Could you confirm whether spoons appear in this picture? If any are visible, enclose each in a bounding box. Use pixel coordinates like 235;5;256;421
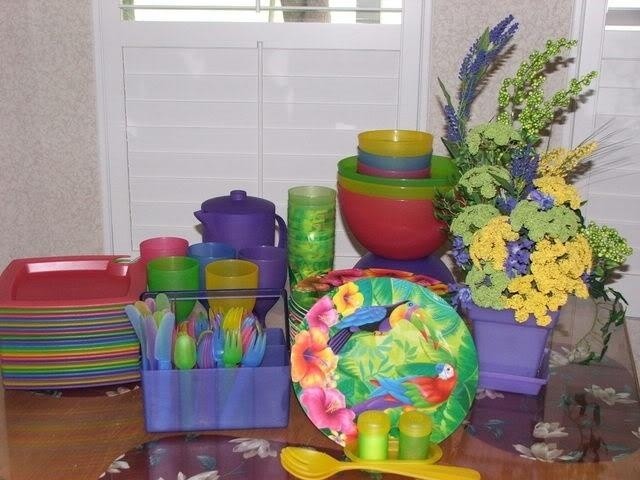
173;333;198;370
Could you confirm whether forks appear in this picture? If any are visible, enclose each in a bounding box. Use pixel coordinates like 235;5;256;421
192;307;269;369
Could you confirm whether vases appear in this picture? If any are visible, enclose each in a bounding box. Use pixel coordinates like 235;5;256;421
462;294;562;399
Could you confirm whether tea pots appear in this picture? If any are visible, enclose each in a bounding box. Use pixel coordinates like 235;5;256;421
194;189;286;247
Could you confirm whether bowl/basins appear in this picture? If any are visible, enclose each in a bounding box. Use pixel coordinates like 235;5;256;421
336;153;464;261
357;129;434;179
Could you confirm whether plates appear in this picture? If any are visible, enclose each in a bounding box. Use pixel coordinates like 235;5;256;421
289;267;461;354
0;253;145;391
289;275;479;449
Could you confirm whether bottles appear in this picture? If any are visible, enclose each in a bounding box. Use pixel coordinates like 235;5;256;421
355;409;391;462
396;408;434;463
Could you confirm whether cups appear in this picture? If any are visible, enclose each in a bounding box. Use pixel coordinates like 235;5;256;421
186;242;234;310
139;237;189;285
287;185;336;291
204;258;259;312
237;244;285;314
146;255;201;320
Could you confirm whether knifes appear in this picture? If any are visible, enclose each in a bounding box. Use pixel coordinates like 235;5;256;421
123;292;175;371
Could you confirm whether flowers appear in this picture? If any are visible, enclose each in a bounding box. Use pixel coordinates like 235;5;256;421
431;16;631;327
